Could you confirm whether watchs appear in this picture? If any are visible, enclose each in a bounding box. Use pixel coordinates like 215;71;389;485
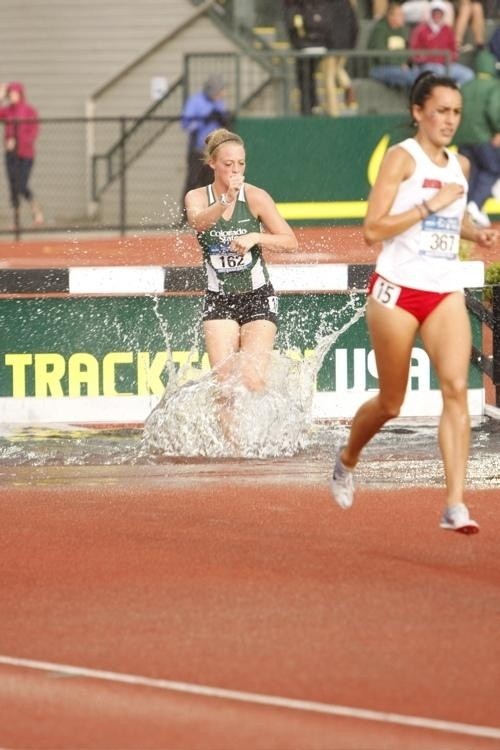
219;191;232;206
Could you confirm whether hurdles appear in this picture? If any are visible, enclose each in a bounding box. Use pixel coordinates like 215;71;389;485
0;260;500;420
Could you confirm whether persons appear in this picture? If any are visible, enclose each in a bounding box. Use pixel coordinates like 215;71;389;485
180;65;238;226
447;51;500;228
284;1;499;119
0;81;45;232
184;130;299;438
329;71;498;534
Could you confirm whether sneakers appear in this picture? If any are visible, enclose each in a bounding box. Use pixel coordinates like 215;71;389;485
438;502;480;536
330;444;355;509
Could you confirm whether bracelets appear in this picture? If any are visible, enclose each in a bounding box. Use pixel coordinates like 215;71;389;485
422;199;436;215
413;204;428;221
